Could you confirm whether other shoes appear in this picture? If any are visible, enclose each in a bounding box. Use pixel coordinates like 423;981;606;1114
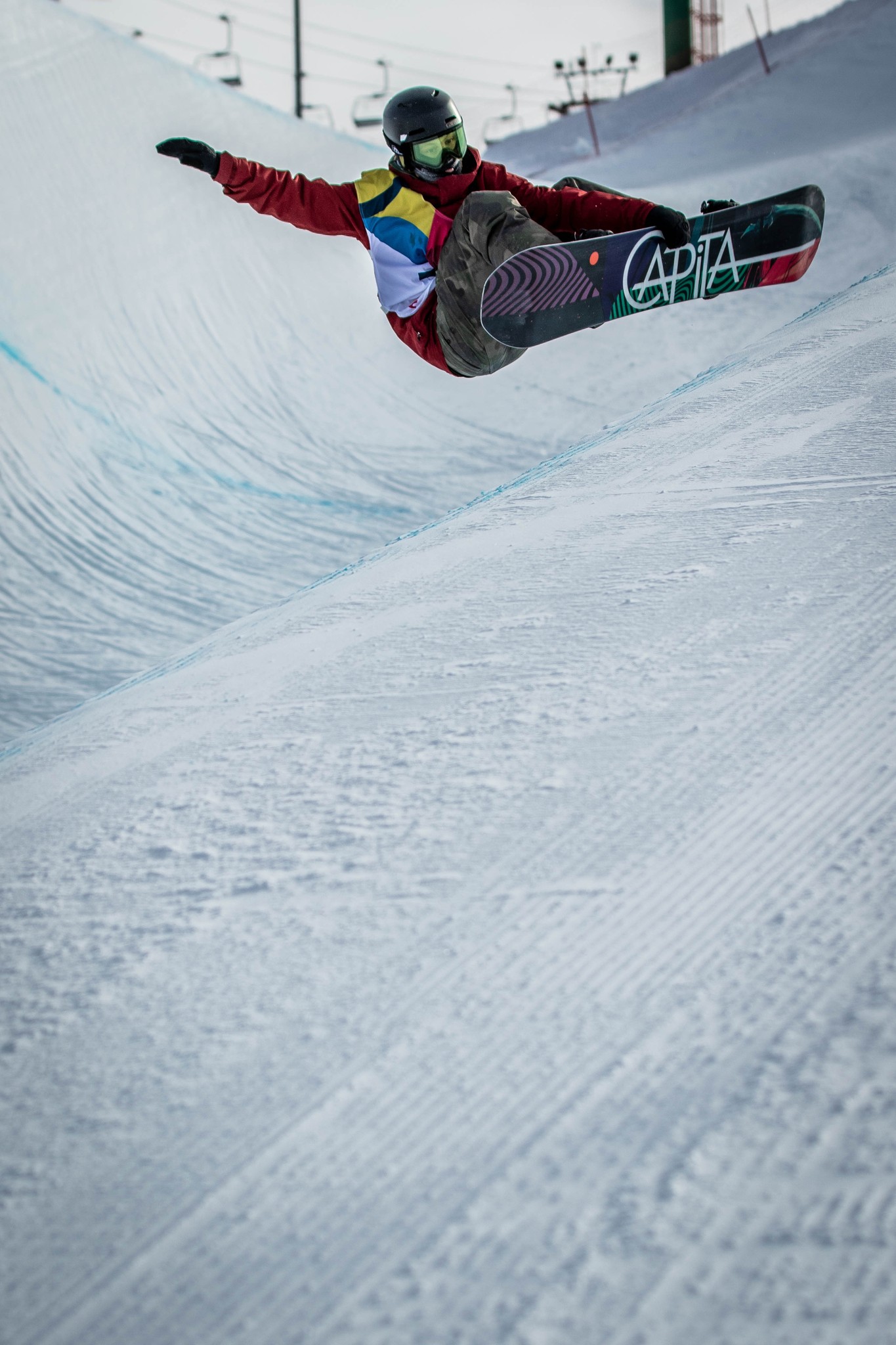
701;199;738;214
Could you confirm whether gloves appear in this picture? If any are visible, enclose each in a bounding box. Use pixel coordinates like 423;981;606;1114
643;206;691;249
156;137;219;178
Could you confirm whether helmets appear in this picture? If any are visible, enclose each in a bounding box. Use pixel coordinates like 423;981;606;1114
382;85;466;180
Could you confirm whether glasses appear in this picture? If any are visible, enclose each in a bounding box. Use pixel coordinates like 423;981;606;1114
407;127;467;169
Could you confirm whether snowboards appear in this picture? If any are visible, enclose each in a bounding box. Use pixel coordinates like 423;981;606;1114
480;184;826;349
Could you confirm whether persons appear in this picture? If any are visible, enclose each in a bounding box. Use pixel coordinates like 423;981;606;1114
156;87;740;379
